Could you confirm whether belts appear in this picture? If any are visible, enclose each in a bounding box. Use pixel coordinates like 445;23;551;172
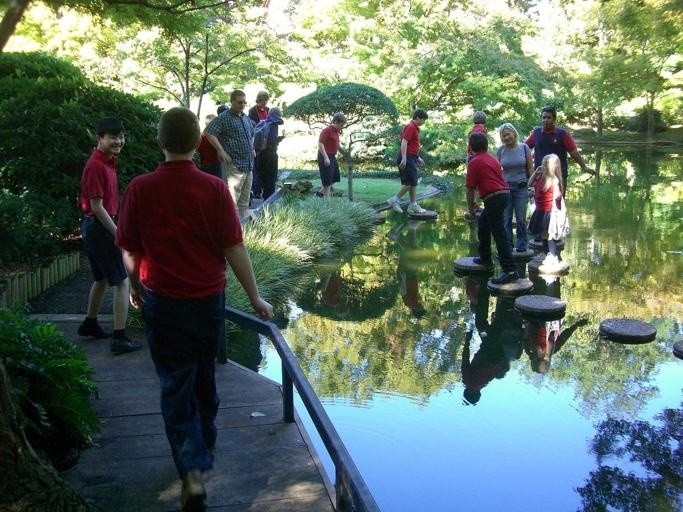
482;190;511;202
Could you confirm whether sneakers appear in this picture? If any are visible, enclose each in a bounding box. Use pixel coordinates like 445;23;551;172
407;205;426;213
111;338;143;353
78;326;108;339
387;197;402;215
531;239;543;246
542;254;560;265
180;470;207;511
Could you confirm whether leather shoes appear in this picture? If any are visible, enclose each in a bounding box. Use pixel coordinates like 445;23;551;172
492;271;520;284
473;256;493;264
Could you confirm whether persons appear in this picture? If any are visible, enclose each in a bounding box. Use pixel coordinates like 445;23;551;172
75;116;144;353
386;109;428;214
115;107;276;512
465;105;596;285
198;90;285;219
315;112;347;198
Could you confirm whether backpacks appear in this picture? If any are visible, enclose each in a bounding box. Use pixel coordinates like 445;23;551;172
253;120;270;151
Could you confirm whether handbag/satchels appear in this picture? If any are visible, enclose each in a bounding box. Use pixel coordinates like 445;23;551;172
548;207;569;241
